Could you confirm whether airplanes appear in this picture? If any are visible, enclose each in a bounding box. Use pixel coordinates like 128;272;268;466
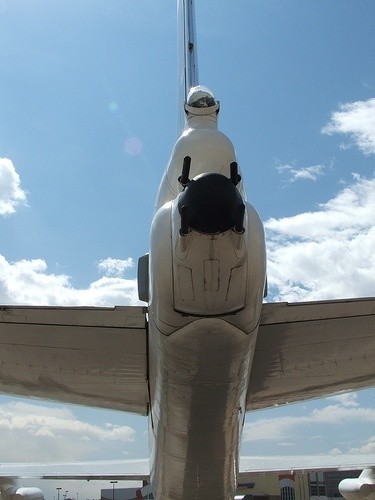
0;84;375;500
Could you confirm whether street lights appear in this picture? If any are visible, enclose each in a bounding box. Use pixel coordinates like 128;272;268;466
110;481;118;500
76;493;79;500
64;490;68;499
56;488;61;500
